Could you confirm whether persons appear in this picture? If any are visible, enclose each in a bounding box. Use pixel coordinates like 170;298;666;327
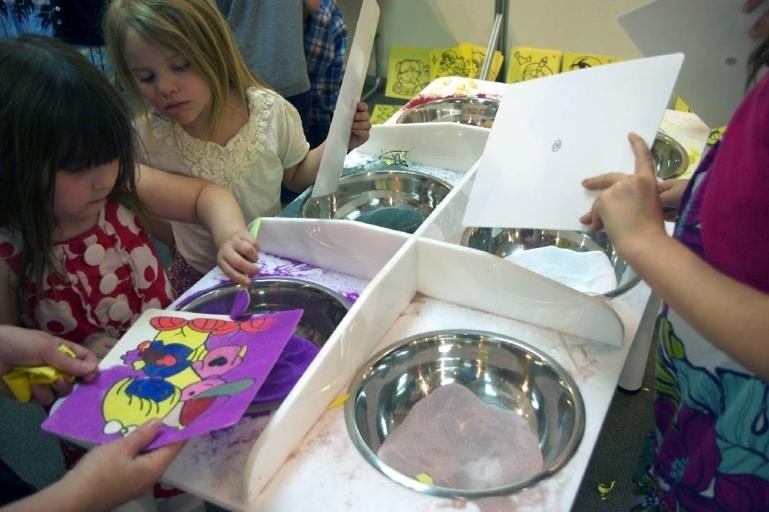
0;321;186;512
2;32;260;381
577;2;767;511
101;2;374;299
279;0;349;204
215;0;323;212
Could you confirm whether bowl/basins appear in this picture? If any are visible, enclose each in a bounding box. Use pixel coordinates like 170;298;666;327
171;277;352;415
346;327;586;496
461;217;647;301
298;169;452;233
635;133;692;180
397;97;500;131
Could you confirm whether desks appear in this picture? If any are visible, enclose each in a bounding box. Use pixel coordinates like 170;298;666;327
49;76;708;512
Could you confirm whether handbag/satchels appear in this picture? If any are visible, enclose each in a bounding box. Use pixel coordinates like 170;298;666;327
50;0;115;46
